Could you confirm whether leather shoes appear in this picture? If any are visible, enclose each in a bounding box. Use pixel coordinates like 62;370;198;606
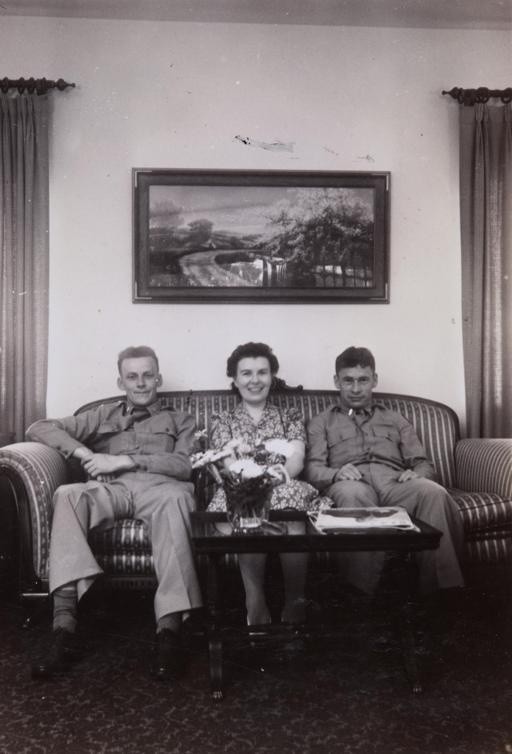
33;625;77;676
152;628;186;679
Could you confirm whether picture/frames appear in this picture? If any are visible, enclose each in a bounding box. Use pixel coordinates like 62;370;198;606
128;165;392;306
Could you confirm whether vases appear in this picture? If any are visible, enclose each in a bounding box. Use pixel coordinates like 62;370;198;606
221;482;273;530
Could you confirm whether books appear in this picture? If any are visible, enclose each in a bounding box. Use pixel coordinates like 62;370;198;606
305;505;421;535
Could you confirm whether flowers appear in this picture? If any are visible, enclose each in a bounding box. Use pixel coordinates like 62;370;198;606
185;421;287;522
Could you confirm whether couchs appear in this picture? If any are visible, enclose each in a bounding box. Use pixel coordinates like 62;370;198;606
1;385;512;628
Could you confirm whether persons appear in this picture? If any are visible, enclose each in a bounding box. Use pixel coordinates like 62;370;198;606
304;345;480;693
24;344;204;682
204;341;310;667
